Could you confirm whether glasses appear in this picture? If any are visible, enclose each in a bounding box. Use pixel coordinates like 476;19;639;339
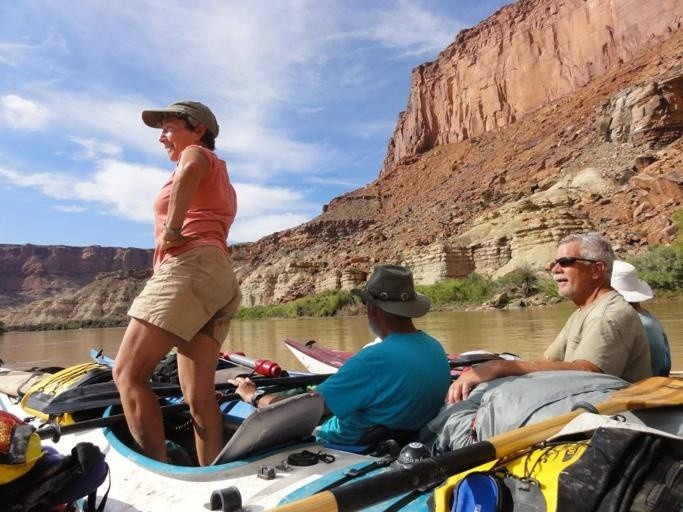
549;256;598;268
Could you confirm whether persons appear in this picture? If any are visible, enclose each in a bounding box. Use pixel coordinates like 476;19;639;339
112;101;242;467
444;232;651;404
227;264;450;454
611;260;672;377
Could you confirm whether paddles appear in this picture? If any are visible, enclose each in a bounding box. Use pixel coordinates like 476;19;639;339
264;377;683;511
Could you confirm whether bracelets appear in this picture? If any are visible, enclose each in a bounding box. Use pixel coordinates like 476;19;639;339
162;225;183;236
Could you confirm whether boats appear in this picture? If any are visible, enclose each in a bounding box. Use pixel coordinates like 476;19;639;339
89;348;378;454
0;368;682;512
284;337;526;382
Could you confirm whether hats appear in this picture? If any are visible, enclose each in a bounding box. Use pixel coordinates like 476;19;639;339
349;265;431;319
610;259;653;303
141;100;219;136
